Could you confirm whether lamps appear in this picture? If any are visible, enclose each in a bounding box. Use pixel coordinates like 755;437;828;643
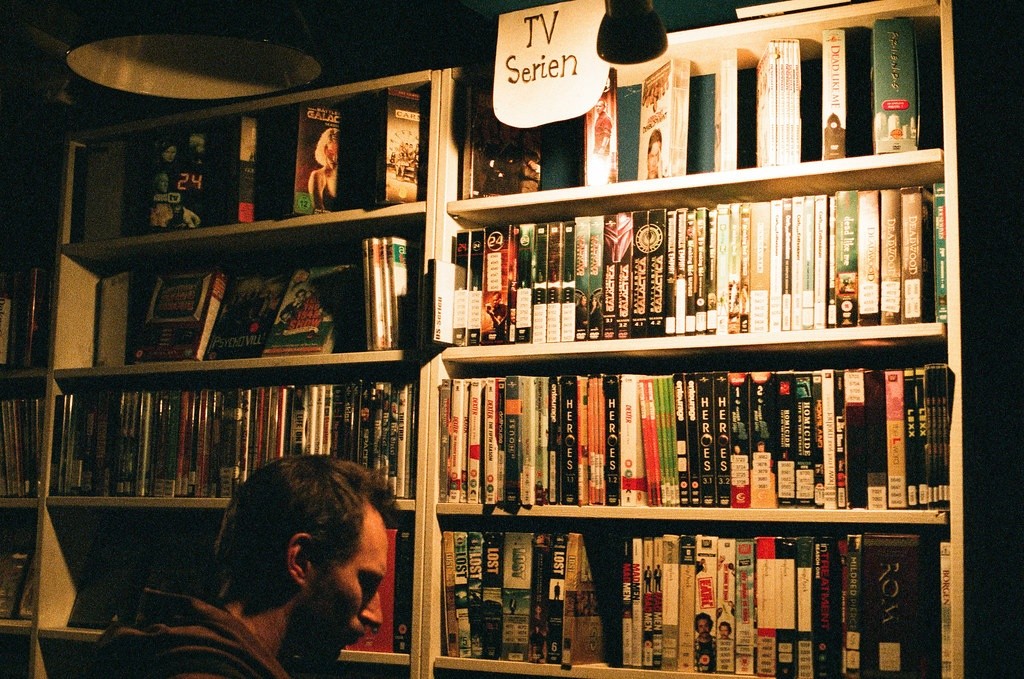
62;0;321;99
596;0;668;65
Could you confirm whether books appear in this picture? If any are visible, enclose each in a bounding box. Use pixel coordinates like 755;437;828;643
438;363;950;509
94;232;418;367
471;67;619;197
125;86;426;231
428;182;948;346
1;267;50;619
715;30;846;170
441;530;952;679
56;379;409;653
638;58;690;180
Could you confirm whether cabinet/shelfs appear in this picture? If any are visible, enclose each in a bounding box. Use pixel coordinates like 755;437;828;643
0;1;967;679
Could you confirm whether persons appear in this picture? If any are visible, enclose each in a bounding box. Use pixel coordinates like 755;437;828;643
308;128;339;212
642;69;670;179
719;622;731;639
644;565;652;593
694;613;716;673
594;102;612;157
83;455;396;679
696;559;707;574
653;565;662;592
554;582;560;599
531;626;544;658
